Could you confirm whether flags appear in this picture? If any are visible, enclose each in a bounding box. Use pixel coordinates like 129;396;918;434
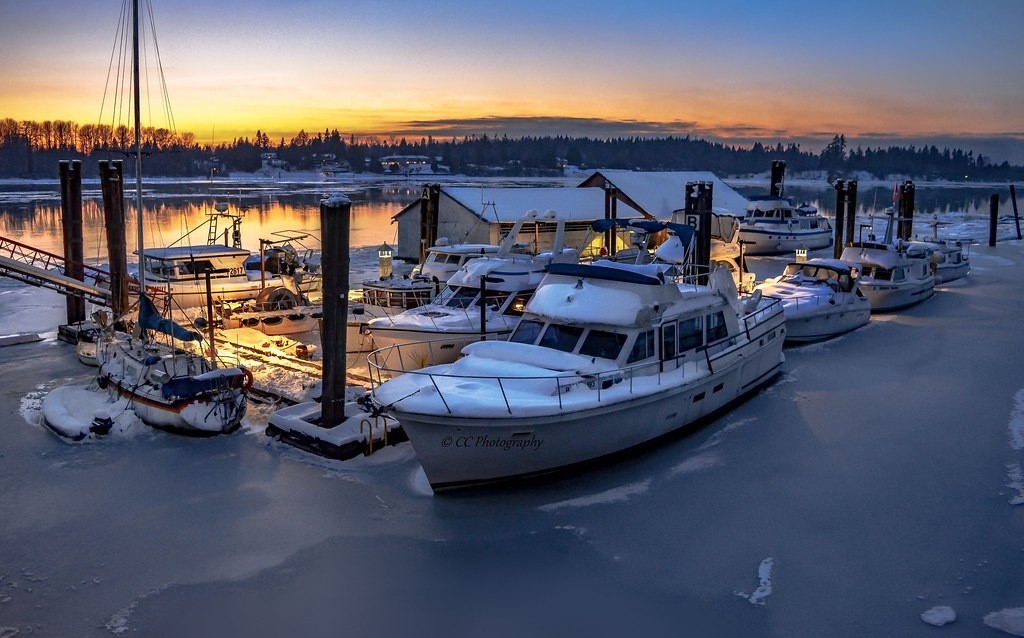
893;183;900;201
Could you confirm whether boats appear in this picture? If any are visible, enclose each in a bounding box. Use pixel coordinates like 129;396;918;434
72;0;976;499
42;384;138;443
